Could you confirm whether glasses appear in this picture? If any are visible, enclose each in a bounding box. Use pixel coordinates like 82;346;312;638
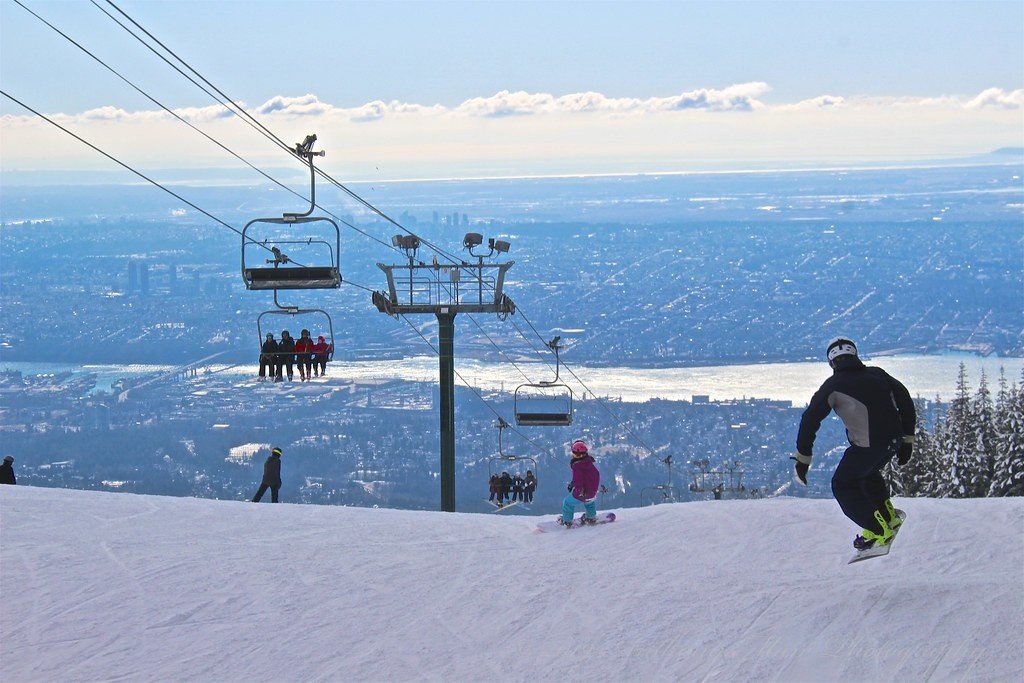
268;336;272;338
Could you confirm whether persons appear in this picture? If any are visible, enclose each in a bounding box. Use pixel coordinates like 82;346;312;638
312;336;331;377
790;337;915;555
0;455;17;484
295;328;315;383
488;473;501;504
257;332;278;383
557;440;600;528
252;447;282;503
510;471;525;505
275;328;297;384
499;471;513;502
523;469;536;505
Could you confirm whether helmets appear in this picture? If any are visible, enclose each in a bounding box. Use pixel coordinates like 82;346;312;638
302;329;309;336
826;335;857;367
318;335;324;343
272;447;282;457
572;441;588;453
282;330;289;337
266;333;273;338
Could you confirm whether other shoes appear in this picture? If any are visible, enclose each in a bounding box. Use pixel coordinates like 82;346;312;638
257;372;324;382
581;514;595;525
555;516;570;527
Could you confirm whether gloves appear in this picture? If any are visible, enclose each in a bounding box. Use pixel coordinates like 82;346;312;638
790;451;813;486
897;435;915;465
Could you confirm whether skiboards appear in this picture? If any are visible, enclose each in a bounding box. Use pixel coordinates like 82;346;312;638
480;497;532;513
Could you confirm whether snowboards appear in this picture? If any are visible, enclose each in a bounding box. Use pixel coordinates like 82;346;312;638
536;510;618;535
847;508;907;565
244;374;331;384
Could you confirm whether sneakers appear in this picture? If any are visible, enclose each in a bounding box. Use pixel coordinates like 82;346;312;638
878;514;899;533
853;528;889;549
